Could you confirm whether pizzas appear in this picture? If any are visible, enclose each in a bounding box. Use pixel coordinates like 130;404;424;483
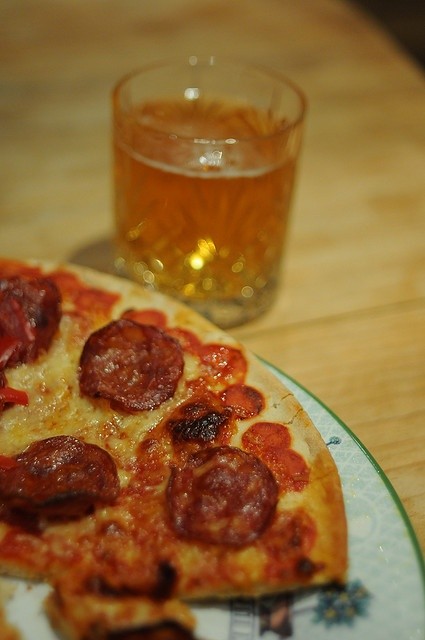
0;253;347;640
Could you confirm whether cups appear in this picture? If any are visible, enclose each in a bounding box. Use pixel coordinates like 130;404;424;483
109;57;308;332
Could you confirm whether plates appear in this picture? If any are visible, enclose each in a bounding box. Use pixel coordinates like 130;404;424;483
2;354;424;640
